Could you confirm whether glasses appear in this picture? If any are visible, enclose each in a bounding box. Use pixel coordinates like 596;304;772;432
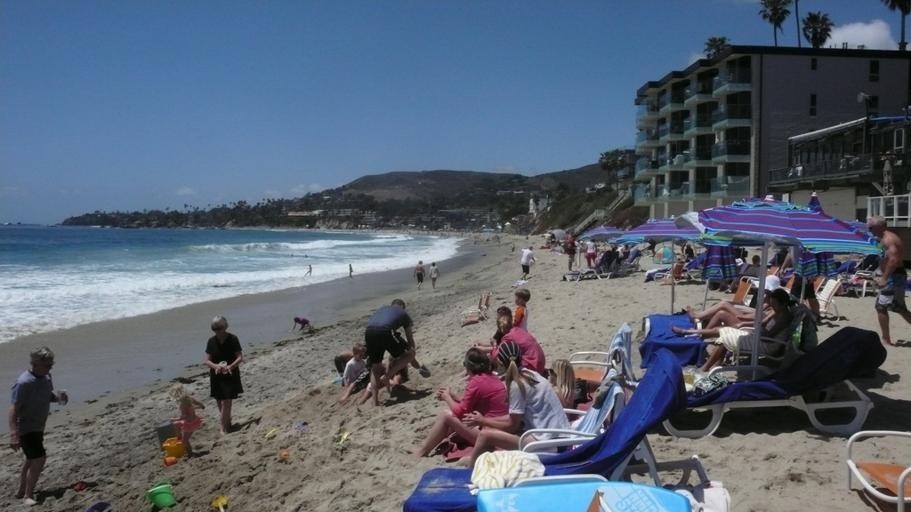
766;264;774;268
867;226;875;231
211;326;221;332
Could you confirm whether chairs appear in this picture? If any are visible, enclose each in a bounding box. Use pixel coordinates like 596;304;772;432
783;274;807;306
401;346;710;512
661;327;888;439
808;276;826;294
729;306;805;376
857;254;881;274
654;260;710;286
843;277;885;299
563;323;634;386
766;266;780;274
712;275;753;309
518;368;627;454
799;279;842;323
563;256;617;283
847;430;911;512
618;249;641;272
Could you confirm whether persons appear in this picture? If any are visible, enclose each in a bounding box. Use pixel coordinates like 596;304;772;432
686;275;788;330
555;240;560;247
414;261;425;289
519;246;535;281
456;340;571;468
492;306;512;347
618;245;630;265
547;358;574;421
512;288;530;329
473;317;546;370
7;346;68;507
671;289;793;371
868;216;911;345
337;333;409;405
641;237;657;257
305;265;312;275
566;234;577;271
205;316;244;433
685;244;694;262
334;351;354;387
169;384;205;462
582;238;597;268
342;344;368;388
716;255;760;293
667;258;684;280
349;264;353;277
409;348;509;458
429;263;440;289
780;237;820;319
293;316;312;332
511;242;516;252
366;299;415;407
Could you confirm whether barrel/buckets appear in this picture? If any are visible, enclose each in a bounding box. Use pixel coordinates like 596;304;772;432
147;481;177;509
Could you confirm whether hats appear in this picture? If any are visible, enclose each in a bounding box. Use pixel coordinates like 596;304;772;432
810;192;819;197
751;274;791;292
